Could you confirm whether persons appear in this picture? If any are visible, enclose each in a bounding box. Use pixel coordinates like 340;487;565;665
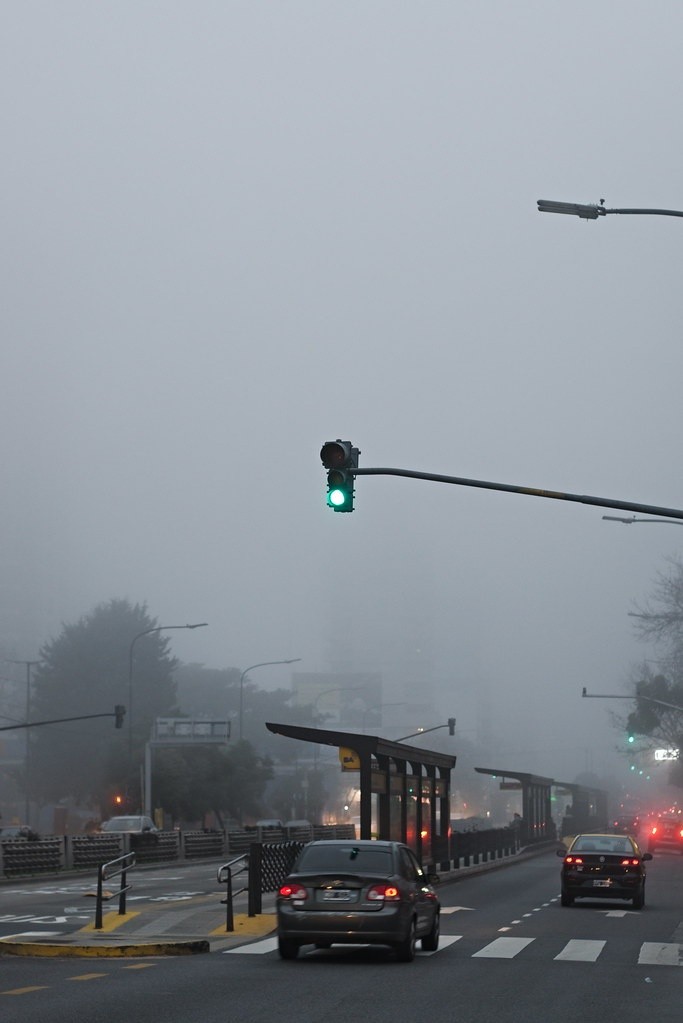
84;816;100;832
511;813;521;824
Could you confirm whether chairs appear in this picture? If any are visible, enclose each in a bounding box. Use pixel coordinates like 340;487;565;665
613;844;626;851
582;842;595;850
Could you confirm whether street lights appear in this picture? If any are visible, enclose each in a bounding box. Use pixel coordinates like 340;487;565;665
10;658;44;823
313;687;363;770
237;659;301;740
125;623;209;812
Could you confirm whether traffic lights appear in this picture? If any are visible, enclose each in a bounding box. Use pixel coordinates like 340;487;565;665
116;704;126;727
114;792;122;806
629;730;634;743
629;758;651;781
319;438;357;514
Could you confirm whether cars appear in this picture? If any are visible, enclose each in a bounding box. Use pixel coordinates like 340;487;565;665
555;831;652;910
609;813;683;855
253;819;315;841
276;840;441;963
94;817;157;832
0;826;38;850
